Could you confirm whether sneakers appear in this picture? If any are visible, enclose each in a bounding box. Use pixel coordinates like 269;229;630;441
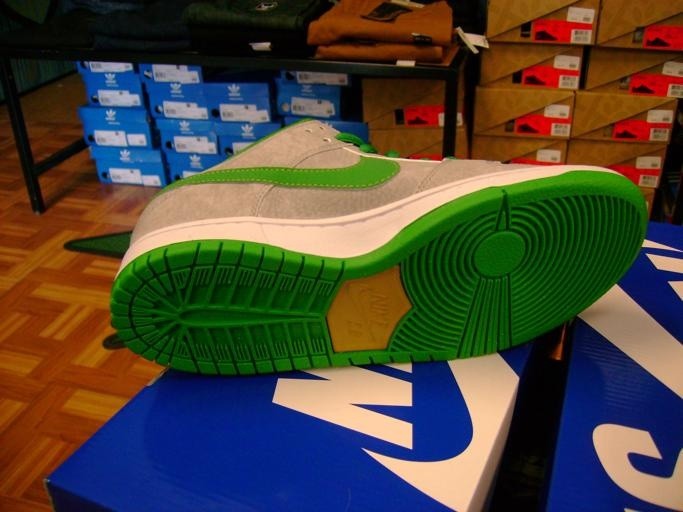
110;119;648;376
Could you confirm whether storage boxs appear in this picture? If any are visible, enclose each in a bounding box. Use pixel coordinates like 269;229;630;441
546;219;683;512
44;322;562;512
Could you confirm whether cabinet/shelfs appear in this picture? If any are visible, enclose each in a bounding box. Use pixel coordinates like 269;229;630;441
0;43;473;214
